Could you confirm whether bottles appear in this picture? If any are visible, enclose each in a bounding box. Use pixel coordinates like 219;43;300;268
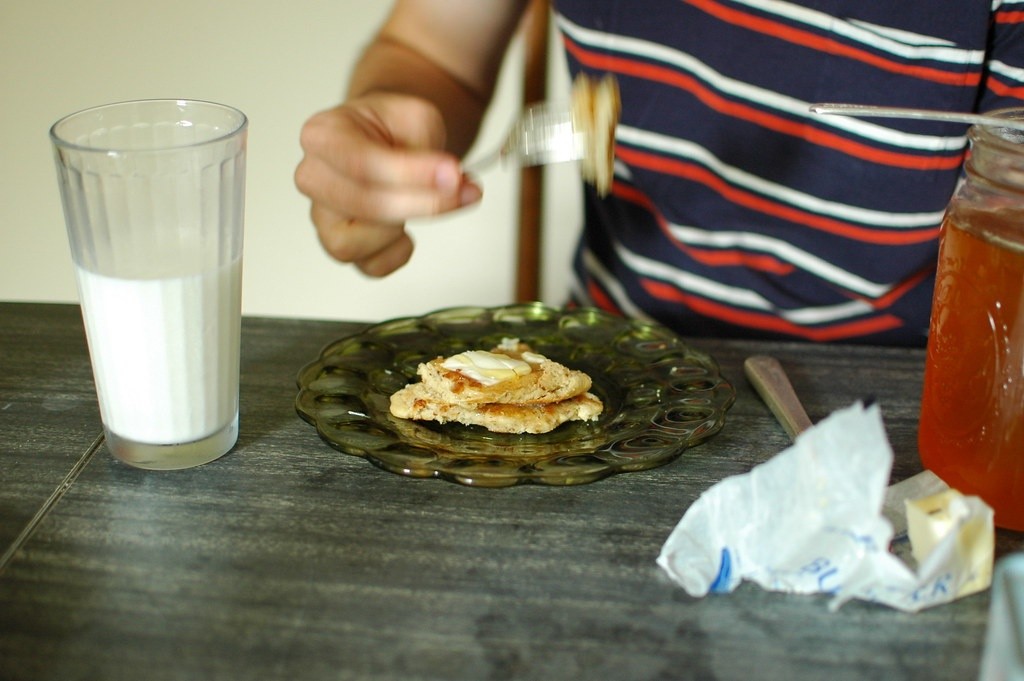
918;106;1024;534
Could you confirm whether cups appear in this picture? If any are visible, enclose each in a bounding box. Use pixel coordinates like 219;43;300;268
49;98;248;472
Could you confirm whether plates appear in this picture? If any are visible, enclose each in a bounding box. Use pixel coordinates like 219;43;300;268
292;301;739;489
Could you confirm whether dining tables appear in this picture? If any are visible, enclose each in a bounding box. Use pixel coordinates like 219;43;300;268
0;302;1023;681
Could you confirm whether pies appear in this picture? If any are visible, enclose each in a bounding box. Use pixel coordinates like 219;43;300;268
575;73;620;193
390;341;604;435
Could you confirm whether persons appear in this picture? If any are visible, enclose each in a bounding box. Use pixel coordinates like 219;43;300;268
295;1;1024;350
